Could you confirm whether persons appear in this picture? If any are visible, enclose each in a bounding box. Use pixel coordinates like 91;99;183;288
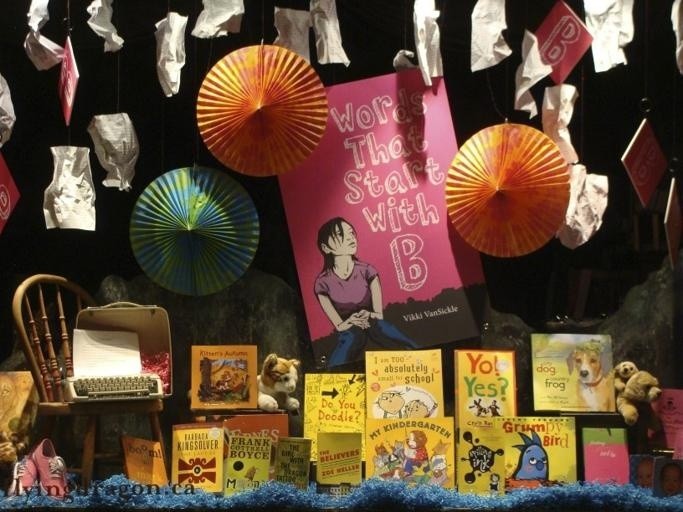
636;457;656;490
660;462;683;498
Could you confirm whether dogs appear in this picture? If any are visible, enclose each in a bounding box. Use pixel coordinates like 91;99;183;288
570;339;616;413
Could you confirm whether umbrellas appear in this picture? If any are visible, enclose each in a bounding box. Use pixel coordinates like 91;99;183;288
196;43;327;177
128;167;259;298
445;123;569;257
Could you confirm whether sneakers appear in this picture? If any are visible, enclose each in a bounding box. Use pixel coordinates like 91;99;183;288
32;439;69;501
8;455;37;497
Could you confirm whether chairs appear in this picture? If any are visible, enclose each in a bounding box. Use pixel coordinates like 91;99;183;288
11;274;168;492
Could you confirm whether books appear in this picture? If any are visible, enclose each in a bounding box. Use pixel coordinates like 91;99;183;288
621;119;667;208
663;177;683;267
58;37;82;129
535;1;594;86
119;334;631;499
0;156;19;240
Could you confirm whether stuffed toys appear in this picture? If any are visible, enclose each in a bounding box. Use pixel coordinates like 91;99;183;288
616;361;660;426
